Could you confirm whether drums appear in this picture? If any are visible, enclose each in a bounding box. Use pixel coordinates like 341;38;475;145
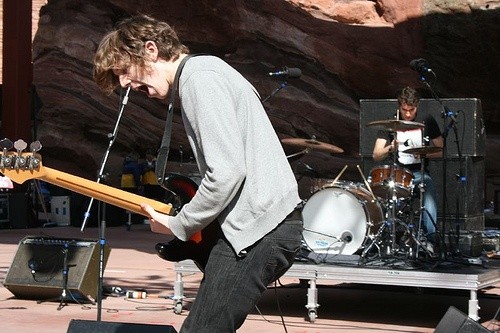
310;178;357;195
301;181;384;256
371;166;414;199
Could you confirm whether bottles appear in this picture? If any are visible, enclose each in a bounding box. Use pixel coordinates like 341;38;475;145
126;291;147;299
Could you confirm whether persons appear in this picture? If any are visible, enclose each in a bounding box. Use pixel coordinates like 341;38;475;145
367;86;443;253
94;15;304;333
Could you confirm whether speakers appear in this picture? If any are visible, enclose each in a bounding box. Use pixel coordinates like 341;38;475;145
1;234;111;303
66;319;178;333
433;305;494;333
428;220;486;260
359;156;484;217
360;99;487;156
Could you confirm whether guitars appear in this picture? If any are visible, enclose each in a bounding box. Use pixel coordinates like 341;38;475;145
0;138;219;273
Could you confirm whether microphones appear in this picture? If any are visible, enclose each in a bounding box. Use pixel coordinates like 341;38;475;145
345;235;352;242
268;67;302;77
409;58;432;74
442;110;458;138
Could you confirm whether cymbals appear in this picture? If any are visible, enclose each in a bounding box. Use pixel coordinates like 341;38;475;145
403;147;445;155
281;138;344;153
369;119;424;131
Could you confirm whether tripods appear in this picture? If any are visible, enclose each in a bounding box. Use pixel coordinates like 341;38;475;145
359;74;473;270
36;243;85;310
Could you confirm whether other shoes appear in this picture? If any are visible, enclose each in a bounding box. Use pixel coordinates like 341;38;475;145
418;243;434;255
